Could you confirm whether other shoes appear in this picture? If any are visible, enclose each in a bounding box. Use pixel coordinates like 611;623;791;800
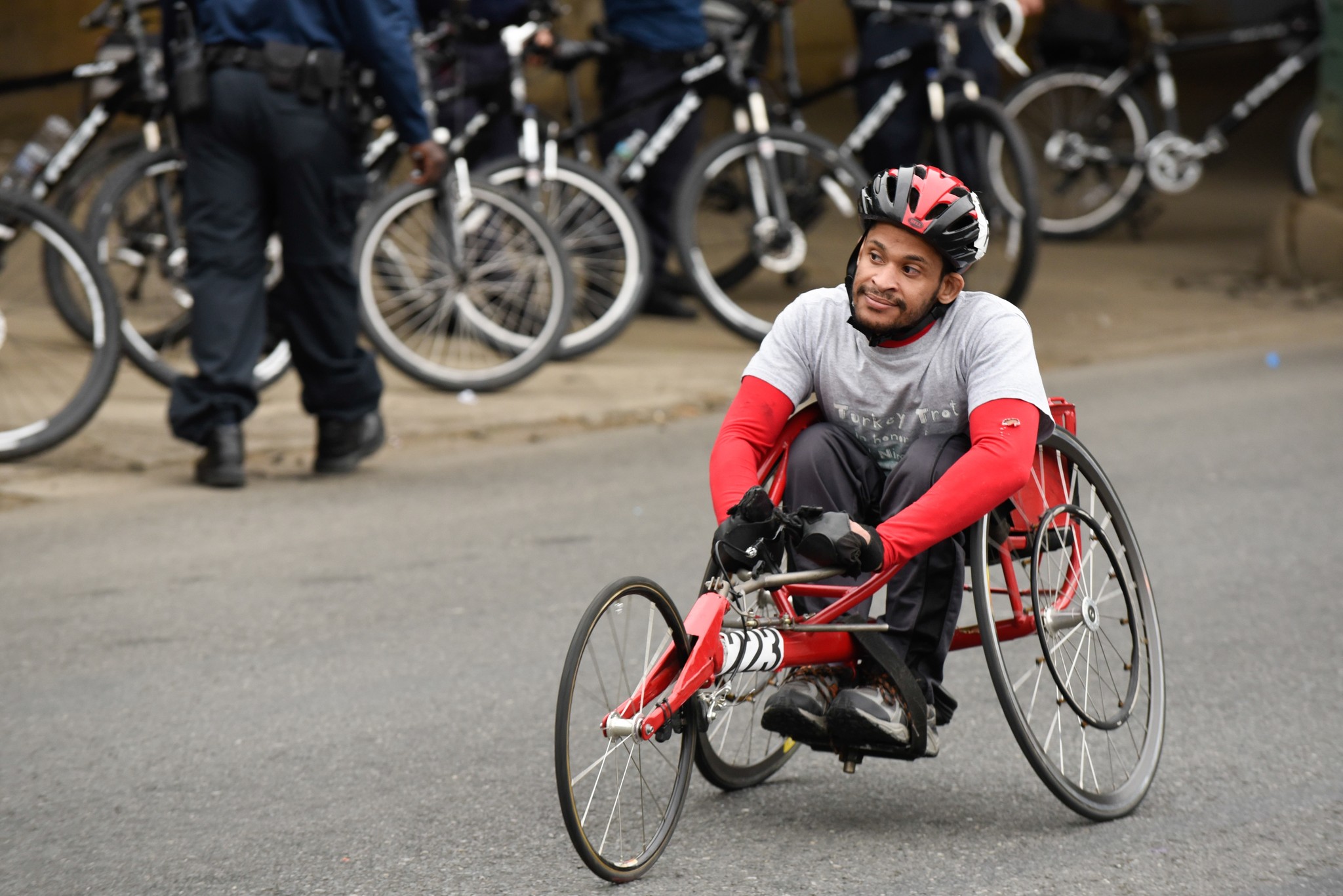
198;426;245;487
642;298;696;319
311;414;386;474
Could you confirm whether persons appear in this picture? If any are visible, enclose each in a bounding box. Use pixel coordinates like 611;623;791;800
599;0;710;318
708;161;1056;759
158;0;447;487
425;0;563;335
848;1;1046;227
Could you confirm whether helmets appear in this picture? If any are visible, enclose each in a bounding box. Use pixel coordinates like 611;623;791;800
855;162;990;275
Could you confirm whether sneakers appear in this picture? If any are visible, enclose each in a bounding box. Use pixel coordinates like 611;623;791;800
758;664;847;752
826;672;940;757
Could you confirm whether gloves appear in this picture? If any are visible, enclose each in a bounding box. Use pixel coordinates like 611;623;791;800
790;505;884;580
709;484;788;571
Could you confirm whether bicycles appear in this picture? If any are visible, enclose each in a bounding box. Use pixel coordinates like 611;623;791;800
984;0;1343;237
1;0;1040;459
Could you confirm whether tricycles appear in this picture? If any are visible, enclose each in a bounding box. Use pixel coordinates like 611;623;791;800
554;393;1172;888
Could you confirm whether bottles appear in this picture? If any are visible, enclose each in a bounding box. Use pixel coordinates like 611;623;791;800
605;128;649;172
